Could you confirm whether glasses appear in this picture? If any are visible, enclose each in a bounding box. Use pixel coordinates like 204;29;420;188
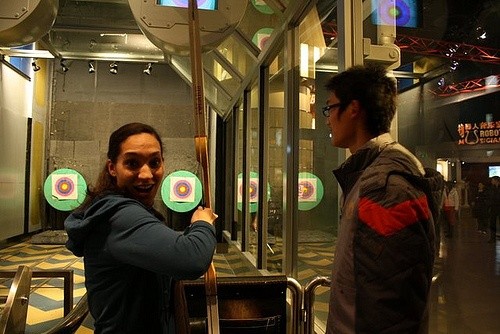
321;102;340;118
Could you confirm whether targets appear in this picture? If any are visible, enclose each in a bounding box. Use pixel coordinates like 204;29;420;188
376;0;411;27
297;177;317;202
53;176;74;197
236;177;259;203
173;180;192;200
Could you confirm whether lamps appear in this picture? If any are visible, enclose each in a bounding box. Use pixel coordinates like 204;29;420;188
109;59;118;75
143;62;152;76
60;59;71;73
31;58;40;71
88;60;96;74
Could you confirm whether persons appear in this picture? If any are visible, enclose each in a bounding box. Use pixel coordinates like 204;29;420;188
63;122;218;334
439;181;459;229
487;176;500;243
471;180;488;235
323;64;442;334
